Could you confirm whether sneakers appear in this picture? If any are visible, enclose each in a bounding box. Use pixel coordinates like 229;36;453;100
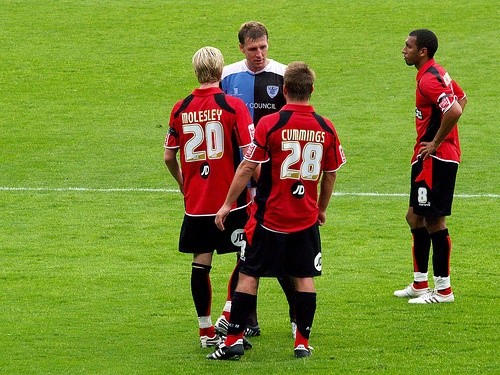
214;314;261;336
292;322;296;339
206;339;244;360
394;284;431;298
199;328;227;347
408;290;454;303
293;344;313;357
215;336;252;350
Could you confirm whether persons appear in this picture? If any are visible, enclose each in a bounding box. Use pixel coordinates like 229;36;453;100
392;28;468;304
219;20;298;340
206;60;348;361
163;45;262;350
